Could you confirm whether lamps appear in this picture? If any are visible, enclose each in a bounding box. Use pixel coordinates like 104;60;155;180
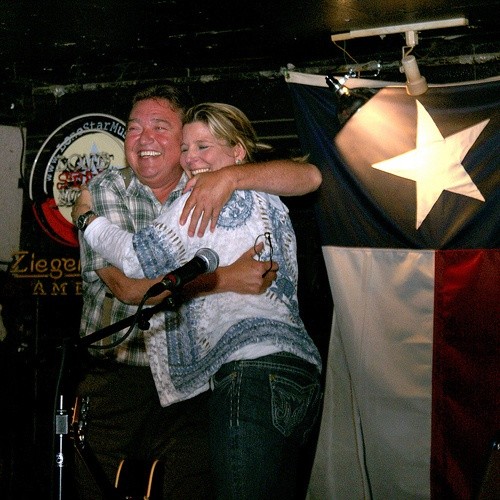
322;76;352;97
400;33;431;97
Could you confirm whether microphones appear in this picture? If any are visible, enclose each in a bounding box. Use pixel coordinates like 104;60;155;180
146;248;219;298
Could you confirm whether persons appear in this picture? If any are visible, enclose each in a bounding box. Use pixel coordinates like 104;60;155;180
69;101;324;499
64;82;281;499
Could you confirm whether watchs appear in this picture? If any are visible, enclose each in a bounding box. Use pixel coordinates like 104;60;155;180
76;211;95;232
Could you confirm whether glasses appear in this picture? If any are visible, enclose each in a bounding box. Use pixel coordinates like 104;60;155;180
253;231;274;279
